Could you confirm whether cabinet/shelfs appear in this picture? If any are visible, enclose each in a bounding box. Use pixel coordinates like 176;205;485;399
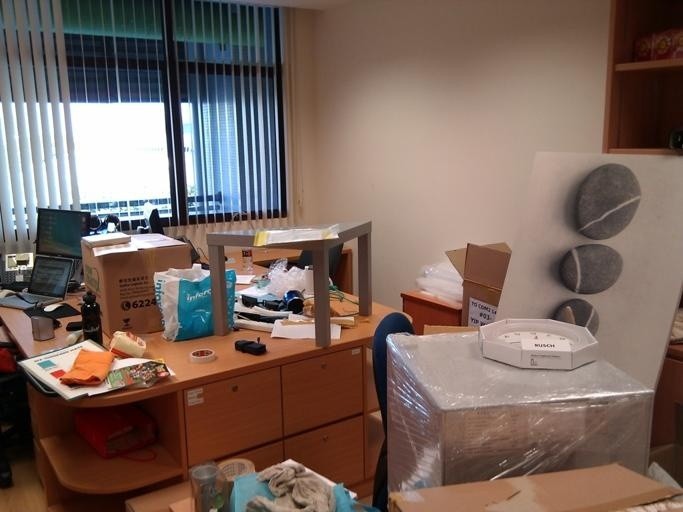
401;284;461;335
601;0;682;155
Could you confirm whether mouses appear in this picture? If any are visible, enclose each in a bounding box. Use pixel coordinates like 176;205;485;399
43;303;64;313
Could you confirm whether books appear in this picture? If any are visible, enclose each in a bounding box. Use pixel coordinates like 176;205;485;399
16;338;121;401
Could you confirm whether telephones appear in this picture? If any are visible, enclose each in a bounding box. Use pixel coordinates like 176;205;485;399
0;253;33;289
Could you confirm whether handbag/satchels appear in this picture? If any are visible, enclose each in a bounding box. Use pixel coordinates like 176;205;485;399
74;403;157;462
153;263;236;342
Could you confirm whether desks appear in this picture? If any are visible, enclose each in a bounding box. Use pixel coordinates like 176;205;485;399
0;249;412;512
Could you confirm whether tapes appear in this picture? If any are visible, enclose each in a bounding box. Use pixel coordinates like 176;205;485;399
190;349;215;363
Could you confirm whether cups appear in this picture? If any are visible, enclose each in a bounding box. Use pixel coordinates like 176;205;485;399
283;289;304;316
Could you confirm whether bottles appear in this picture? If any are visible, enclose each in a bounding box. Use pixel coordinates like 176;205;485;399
240;247;253;271
79;290;102;345
189;465;219;512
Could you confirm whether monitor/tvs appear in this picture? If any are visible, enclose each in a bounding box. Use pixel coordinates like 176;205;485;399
35;207;91;290
136;202;165;235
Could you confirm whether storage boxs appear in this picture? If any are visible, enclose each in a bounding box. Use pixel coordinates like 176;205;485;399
382;458;682;512
80;232;191;340
443;239;511;327
125;478;203;512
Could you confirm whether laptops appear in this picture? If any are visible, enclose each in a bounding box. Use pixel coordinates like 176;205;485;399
0;255;74;311
